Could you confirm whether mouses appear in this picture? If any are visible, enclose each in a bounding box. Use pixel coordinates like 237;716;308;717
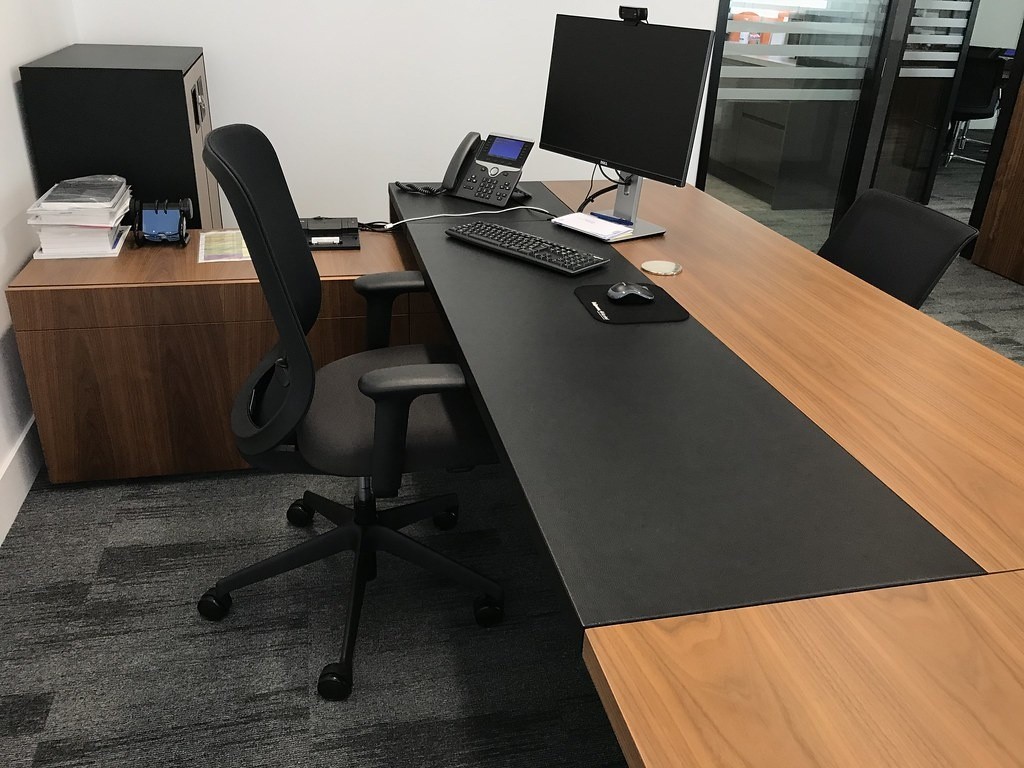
607;282;655;305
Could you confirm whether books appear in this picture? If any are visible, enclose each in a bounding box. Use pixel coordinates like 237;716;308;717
551;212;633;242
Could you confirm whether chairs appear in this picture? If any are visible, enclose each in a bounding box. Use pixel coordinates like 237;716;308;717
816;187;980;312
940;57;1006;168
198;123;519;702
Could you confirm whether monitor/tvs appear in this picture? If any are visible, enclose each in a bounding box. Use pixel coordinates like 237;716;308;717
538;14;715;242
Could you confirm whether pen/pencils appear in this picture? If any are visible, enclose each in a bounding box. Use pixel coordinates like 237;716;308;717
590;211;634;226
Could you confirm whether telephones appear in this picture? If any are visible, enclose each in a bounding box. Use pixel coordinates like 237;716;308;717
441;131;536;208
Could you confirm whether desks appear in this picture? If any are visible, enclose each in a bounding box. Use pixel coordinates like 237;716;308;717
709;56;863;211
388;177;1024;768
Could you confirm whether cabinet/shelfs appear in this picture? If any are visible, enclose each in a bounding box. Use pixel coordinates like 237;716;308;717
6;228;494;491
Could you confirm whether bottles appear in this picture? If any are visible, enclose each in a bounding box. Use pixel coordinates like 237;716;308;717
739;18;760;45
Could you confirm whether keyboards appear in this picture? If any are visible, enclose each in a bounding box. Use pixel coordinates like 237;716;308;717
445;220;611;275
